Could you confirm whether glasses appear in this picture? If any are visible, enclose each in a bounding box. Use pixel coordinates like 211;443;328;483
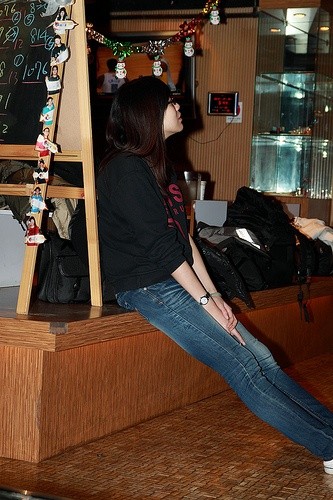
166;94;177;106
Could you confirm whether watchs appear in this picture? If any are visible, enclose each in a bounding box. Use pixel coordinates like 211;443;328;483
199;292;210;307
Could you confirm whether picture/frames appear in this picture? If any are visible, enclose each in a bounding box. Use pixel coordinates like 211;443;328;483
207;91;239;117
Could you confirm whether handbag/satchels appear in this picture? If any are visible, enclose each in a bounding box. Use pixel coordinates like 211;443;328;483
34;230;92;306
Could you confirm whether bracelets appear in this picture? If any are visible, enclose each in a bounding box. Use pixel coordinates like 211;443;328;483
211;292;222;299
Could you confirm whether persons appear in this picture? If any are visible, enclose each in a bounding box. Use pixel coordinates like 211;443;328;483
65;76;333;477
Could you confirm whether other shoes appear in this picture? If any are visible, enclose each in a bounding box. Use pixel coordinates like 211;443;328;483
322;458;333;477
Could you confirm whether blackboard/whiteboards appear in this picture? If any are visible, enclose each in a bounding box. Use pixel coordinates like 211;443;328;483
0;0;72;158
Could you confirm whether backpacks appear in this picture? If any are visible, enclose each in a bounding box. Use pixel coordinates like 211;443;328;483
189;186;333;323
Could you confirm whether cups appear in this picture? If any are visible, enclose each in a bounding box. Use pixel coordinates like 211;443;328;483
200;181;206;201
